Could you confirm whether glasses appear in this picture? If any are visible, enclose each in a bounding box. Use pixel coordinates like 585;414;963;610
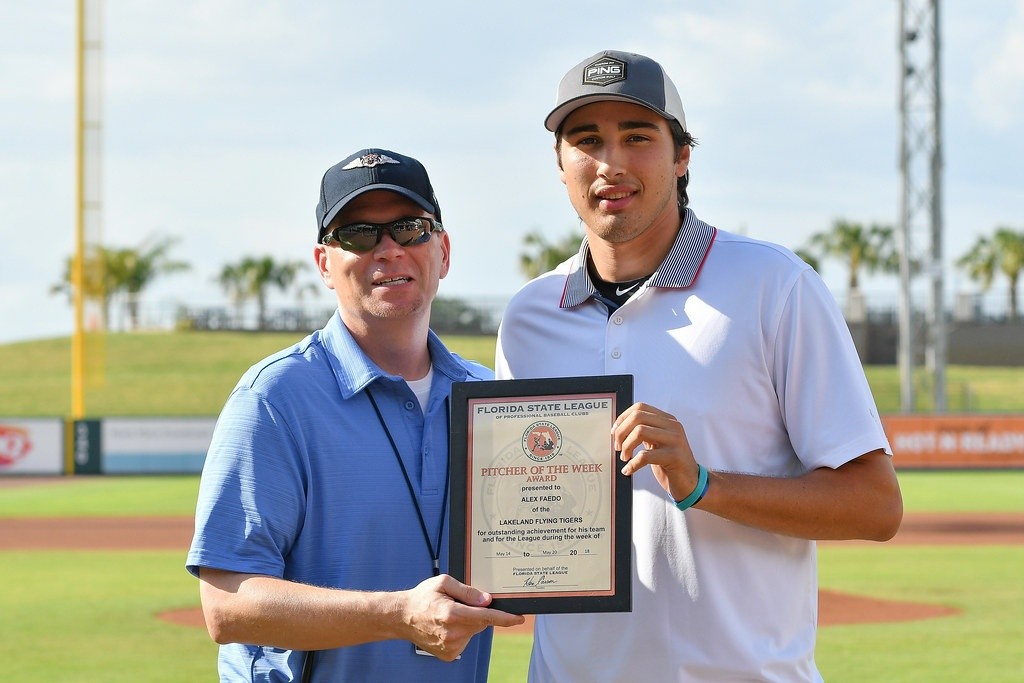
322;216;442;251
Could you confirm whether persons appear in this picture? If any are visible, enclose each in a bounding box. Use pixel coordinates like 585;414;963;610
185;148;526;683
494;51;902;683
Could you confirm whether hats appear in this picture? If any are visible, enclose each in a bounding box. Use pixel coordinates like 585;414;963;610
544;50;687;135
316;149;441;245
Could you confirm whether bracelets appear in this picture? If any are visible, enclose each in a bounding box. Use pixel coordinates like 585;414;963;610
676;464;710;511
